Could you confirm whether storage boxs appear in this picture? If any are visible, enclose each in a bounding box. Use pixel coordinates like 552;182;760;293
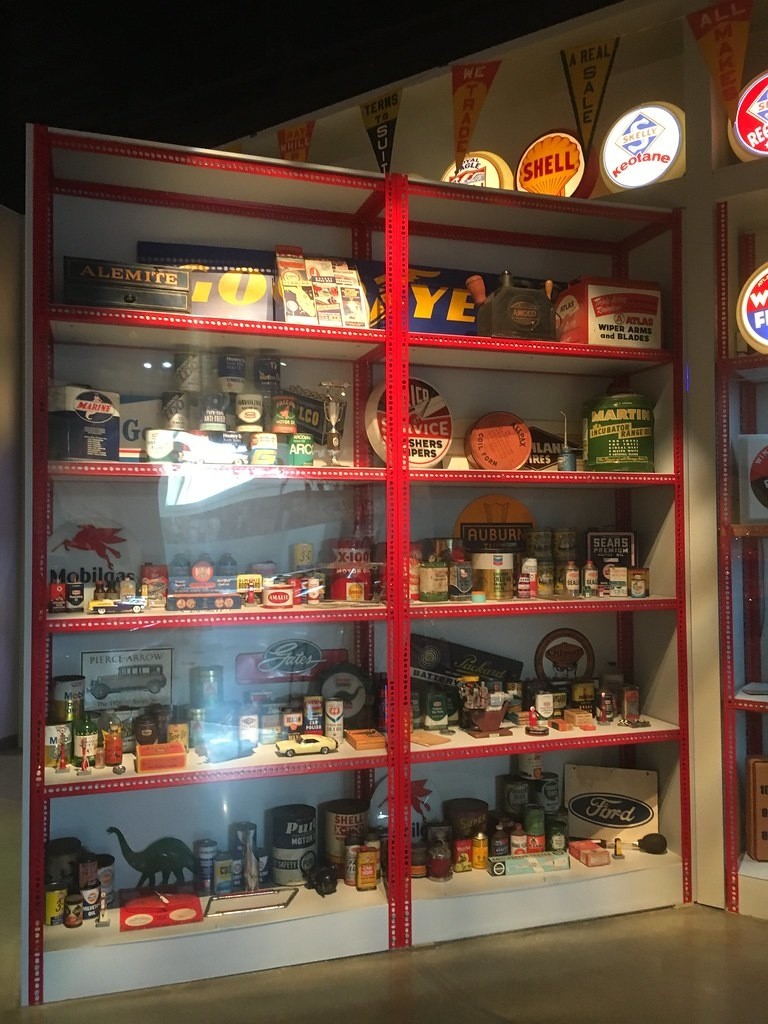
135;741;189;774
48;385;121;462
738;435;768;525
563;709;593;725
745;753;768;861
61;256;193;312
555;279;664;351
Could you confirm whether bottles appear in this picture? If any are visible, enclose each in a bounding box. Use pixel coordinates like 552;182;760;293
510;823;527;856
217;553;237;575
582;560;598;596
600;661;624;715
170;553;191;577
474;829;489;870
562;560;580;600
104;580;119;600
119;574;136;599
344;828;363;887
491;822;508;856
363;824;381;883
197;553;214;578
73;713;99;767
94;580;105;601
104;724;122;767
199;838;217;893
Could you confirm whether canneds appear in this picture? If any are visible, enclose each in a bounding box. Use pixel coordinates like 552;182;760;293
147;351;315;467
433;752;570;853
45;797;383;929
409;529;650;602
46;665;344;769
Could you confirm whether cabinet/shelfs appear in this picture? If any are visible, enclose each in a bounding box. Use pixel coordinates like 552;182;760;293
23;124;696;1012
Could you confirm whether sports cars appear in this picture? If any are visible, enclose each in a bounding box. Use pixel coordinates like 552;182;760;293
276;734;337;758
